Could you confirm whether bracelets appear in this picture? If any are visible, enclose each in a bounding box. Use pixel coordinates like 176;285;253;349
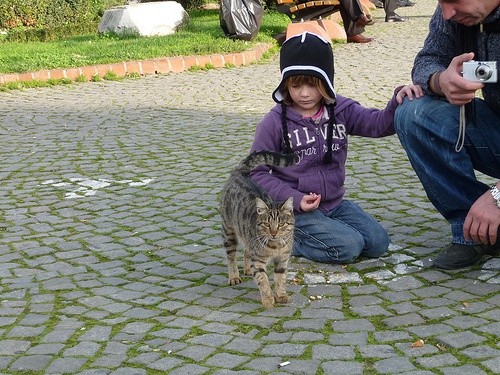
434;71;443;96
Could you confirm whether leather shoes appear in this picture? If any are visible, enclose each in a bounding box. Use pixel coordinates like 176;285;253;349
385;12;407;22
355;15;374;25
347;33;374;43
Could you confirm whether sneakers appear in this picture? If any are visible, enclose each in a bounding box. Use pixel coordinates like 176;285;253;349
433;241;500;269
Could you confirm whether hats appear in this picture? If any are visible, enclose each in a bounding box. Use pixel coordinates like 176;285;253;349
272;31;337;167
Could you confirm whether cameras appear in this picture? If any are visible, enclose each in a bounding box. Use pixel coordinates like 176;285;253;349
463;61;498;83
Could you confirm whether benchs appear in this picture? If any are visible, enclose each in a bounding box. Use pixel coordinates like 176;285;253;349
276;0;347;46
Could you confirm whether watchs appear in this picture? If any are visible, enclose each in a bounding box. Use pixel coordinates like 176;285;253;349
490;183;500;208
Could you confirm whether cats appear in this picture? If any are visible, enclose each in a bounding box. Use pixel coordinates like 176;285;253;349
222;149;302;309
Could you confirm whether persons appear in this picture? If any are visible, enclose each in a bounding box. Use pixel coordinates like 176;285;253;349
297;0;376;42
394;0;500;270
381;0;415;22
249;32;423;262
